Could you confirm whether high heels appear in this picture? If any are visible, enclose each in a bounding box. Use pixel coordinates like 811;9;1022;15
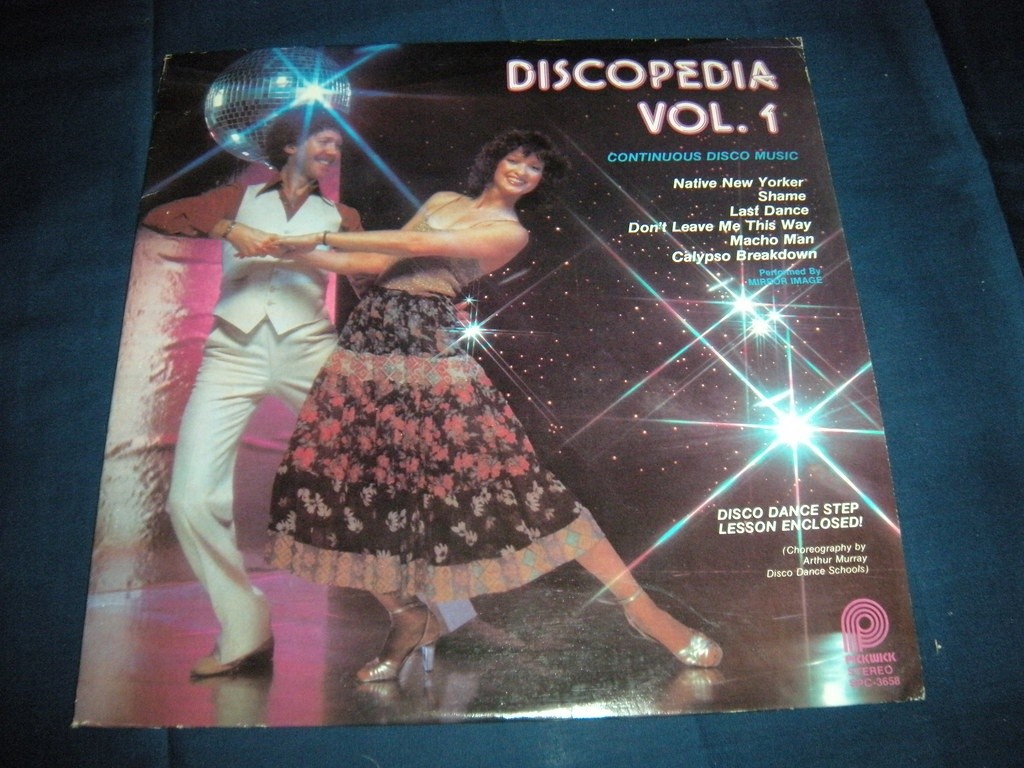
357;601;440;684
618;587;724;667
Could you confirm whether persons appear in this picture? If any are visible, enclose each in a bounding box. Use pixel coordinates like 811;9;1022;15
143;105;525;676
233;133;724;683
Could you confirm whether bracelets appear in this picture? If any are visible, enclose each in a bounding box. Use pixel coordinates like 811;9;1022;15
222;220;236;238
323;230;330;245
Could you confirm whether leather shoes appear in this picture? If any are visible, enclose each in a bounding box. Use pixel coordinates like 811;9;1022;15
191;633;276;676
456;615;525;653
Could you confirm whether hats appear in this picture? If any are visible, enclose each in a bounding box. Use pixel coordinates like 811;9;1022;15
268;105;353;167
